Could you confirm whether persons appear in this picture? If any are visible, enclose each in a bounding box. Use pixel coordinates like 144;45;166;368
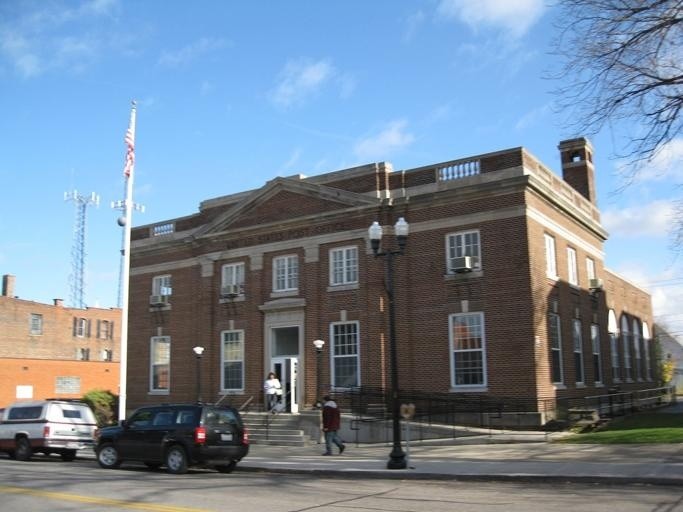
263;373;282;413
320;396;345;456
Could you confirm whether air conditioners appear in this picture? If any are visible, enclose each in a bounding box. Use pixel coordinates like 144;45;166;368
589;279;603;289
220;283;238;297
149;295;169;308
450;257;473;273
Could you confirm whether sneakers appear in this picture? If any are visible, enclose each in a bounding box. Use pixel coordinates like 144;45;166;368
339;444;345;453
321;449;332;457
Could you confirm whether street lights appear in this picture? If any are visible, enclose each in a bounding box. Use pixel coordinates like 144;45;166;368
313;339;324;410
366;217;408;469
191;346;204;405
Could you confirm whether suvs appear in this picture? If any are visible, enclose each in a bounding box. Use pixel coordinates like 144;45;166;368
0;399;97;460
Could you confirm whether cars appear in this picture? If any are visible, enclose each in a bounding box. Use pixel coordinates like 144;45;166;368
93;403;249;475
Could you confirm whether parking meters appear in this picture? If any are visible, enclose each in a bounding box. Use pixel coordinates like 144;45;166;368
399;404;416;469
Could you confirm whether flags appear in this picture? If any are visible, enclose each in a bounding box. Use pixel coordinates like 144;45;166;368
123;119;136;177
